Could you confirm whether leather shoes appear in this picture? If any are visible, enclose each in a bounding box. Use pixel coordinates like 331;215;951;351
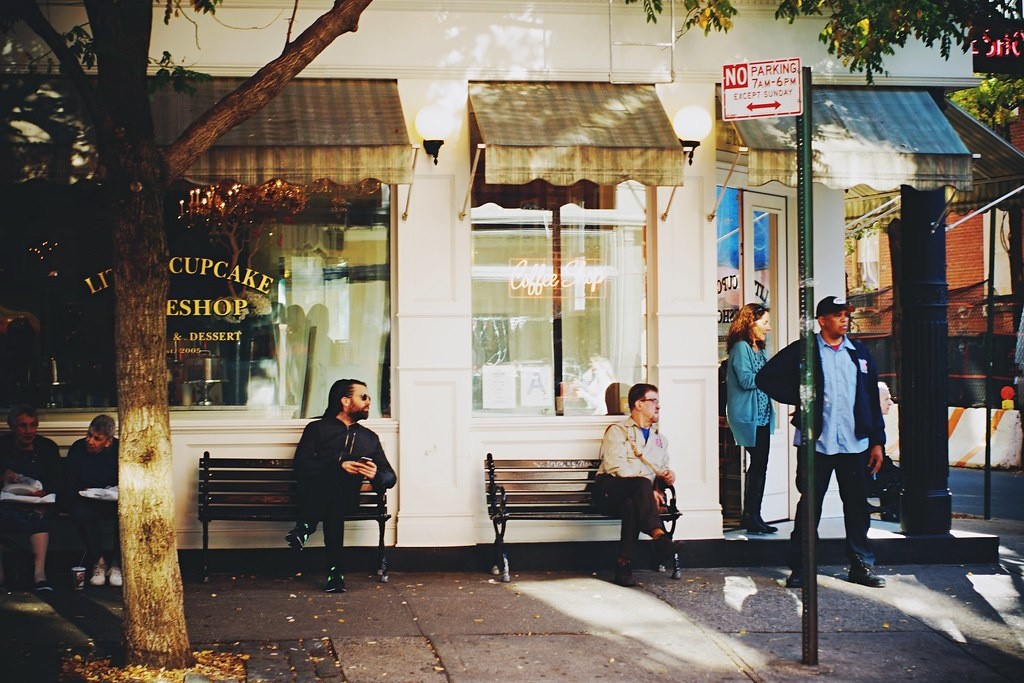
787;567;804;586
847;552;885;587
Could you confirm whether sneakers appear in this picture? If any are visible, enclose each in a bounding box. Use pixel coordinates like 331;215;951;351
106;565;123;586
90;563;107;585
325;562;345;592
286;522;309;549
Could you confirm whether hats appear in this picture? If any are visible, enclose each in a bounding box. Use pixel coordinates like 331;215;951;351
813;296;847;319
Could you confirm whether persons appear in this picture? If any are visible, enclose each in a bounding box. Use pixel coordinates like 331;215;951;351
754;296;886;586
0;403;64;590
725;304;779;533
286;379;396;591
867;382;900;522
594;384;684;587
66;415;123;586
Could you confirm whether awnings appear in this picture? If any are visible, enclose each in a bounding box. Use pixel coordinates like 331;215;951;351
710;88;975;231
462;81;684;222
846;96;1024;232
145;78;414;186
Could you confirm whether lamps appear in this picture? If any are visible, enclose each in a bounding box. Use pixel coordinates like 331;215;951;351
416;106;454;165
669;104;713;164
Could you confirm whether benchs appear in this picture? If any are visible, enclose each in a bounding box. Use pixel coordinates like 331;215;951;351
484;453;681;582
197;451;392;567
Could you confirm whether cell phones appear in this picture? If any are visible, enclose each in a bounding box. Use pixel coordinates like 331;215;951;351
357;457;373;465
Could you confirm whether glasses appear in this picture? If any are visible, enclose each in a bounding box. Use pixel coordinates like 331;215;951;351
639;399;659;405
346;393;371;402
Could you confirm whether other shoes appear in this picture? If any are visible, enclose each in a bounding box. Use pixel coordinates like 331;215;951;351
655;534;685;563
741;514;779;533
34;579;53;590
616;560;636;585
